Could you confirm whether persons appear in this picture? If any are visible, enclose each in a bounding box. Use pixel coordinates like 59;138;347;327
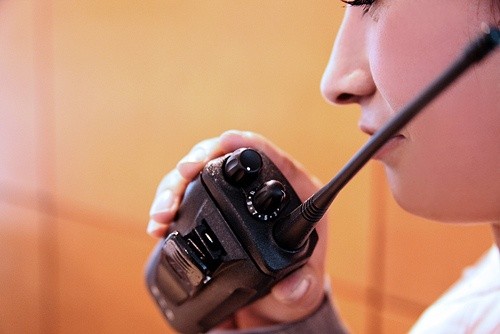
147;0;500;334
